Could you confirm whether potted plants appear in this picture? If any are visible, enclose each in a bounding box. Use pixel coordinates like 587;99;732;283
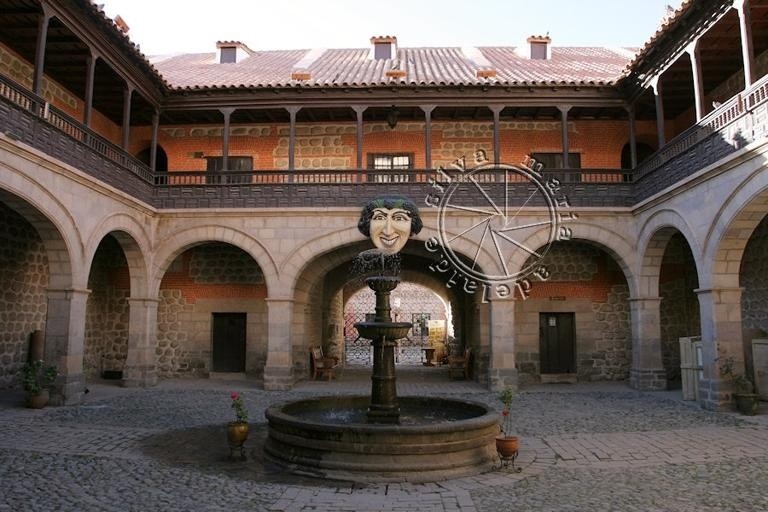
713;343;760;416
16;360;58;408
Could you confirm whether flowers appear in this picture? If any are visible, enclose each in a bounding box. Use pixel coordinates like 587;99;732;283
230;391;248;424
498;385;512;439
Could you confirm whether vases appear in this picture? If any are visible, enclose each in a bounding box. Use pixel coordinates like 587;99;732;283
491;435;523;474
227;421;249;462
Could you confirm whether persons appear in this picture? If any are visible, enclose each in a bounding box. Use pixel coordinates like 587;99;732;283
357;198;424;256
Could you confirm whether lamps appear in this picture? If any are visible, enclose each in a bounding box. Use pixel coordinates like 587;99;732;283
386;108;401;129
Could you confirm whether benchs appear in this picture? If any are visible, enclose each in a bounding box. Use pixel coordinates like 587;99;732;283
447;347;471;380
310;346;336;382
